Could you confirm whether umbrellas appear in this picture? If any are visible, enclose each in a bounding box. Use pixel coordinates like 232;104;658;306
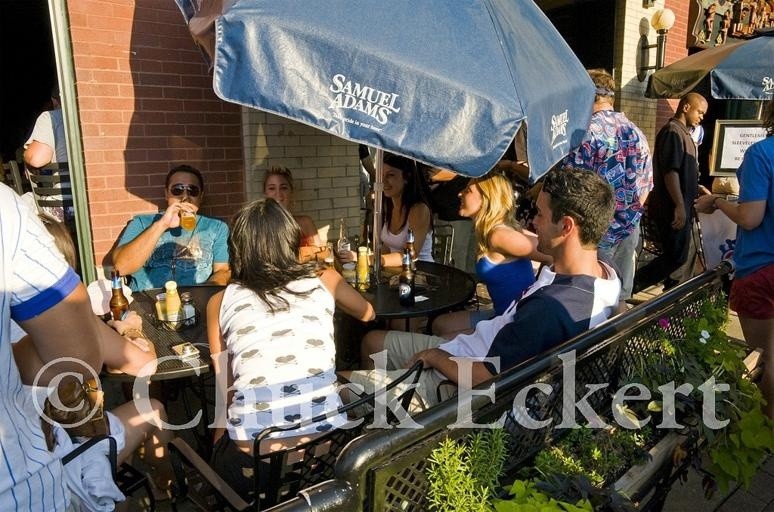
644;27;774;120
173;0;597;284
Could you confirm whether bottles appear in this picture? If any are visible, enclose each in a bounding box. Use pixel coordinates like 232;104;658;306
109;271;130;321
343;246;373;292
325;242;335;270
155;279;196;329
399;227;419;305
337;218;351;260
180;199;198;229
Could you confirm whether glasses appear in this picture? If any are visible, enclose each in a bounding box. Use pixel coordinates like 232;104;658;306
167;183;202;198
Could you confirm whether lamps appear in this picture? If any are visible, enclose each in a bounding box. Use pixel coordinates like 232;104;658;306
635;8;675;82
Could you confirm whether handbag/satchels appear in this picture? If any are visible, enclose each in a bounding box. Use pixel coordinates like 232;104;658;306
41;375;111;452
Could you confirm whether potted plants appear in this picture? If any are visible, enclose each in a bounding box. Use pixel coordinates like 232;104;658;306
536;285;774;512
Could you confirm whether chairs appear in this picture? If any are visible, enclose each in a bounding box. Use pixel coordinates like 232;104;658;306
636;203;664;272
166;360;425;511
0;159;24;196
691;192;740;271
437;299;646;403
368;223;455;265
60;433;156;512
24;160;76;226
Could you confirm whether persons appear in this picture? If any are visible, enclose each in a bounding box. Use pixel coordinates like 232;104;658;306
631;92;709;295
712;176;740;197
705;3;716;42
693;94;774;420
0;68;654;512
721;10;731;45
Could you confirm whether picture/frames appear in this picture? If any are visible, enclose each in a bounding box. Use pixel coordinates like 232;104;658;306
709;119;774;177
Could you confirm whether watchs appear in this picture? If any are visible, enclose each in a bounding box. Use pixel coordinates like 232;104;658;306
712;197;724;209
